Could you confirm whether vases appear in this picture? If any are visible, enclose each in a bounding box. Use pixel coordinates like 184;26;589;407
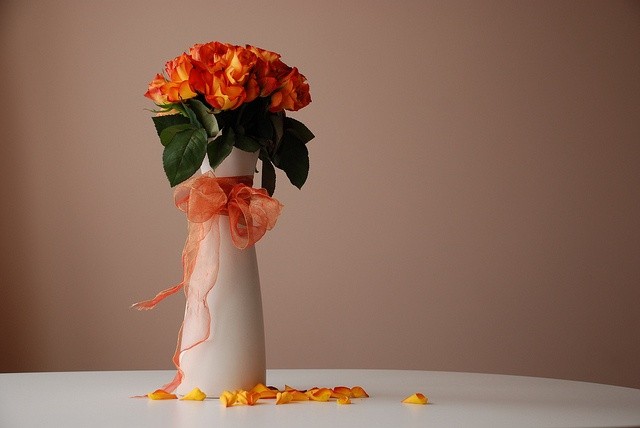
179;135;267;400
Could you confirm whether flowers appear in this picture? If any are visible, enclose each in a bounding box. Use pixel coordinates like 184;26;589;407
139;40;316;201
